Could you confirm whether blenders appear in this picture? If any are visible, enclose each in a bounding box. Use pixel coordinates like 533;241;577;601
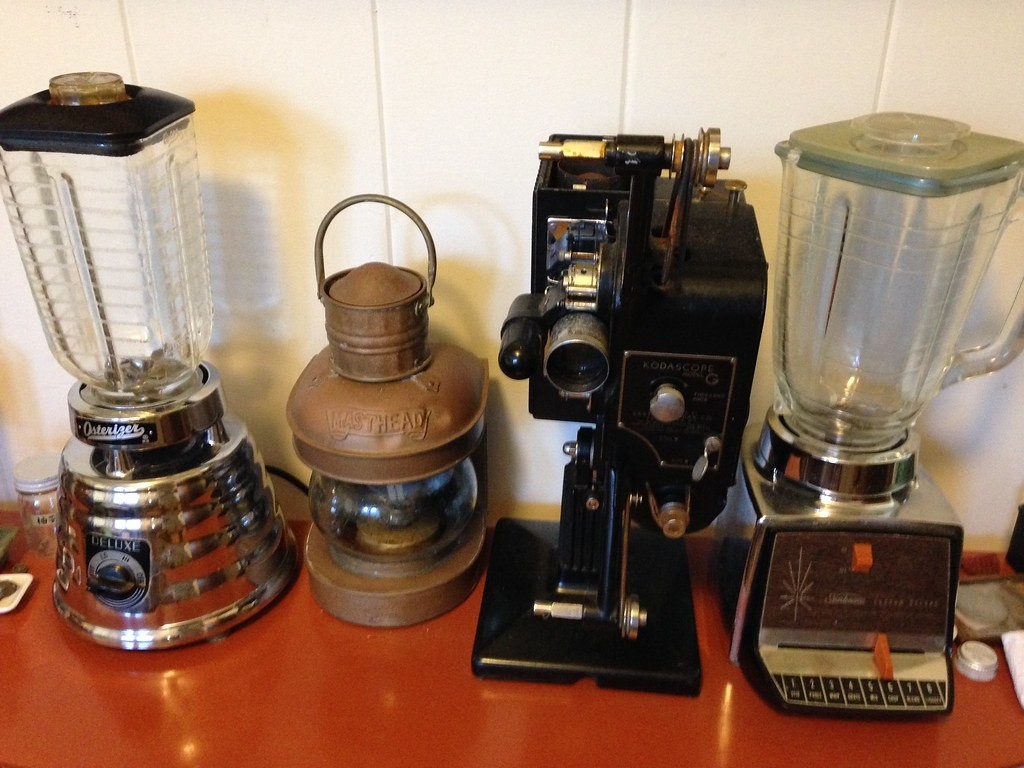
711;110;1023;719
0;74;299;651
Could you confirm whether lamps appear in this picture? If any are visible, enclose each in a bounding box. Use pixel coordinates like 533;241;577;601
280;193;490;628
